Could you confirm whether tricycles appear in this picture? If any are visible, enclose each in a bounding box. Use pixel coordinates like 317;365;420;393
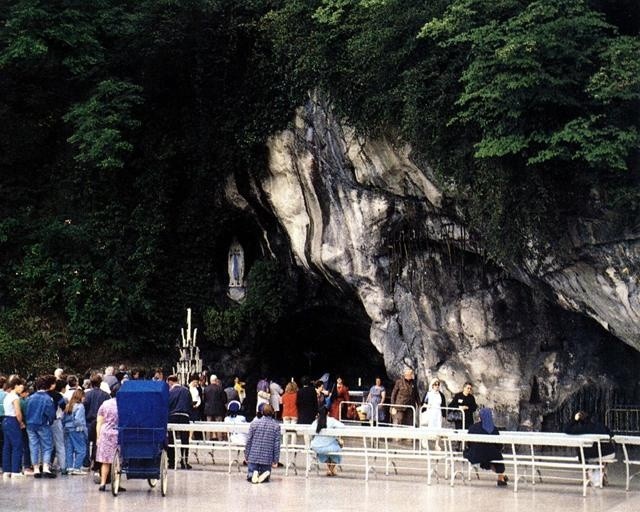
109;377;171;499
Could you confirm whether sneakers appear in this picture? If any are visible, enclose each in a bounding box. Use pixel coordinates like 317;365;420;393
252;470;258;483
258;470;270;483
498;475;508;486
93;470;126;492
0;467;91;478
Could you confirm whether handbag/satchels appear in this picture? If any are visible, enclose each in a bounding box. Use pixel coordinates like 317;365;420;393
258;390;270;400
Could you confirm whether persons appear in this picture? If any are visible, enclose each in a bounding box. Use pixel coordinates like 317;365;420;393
463;407;508;489
448;380;478;452
563;409;617;488
365;376;387;425
422;377;446;451
390;368;422;446
0;363;356;492
